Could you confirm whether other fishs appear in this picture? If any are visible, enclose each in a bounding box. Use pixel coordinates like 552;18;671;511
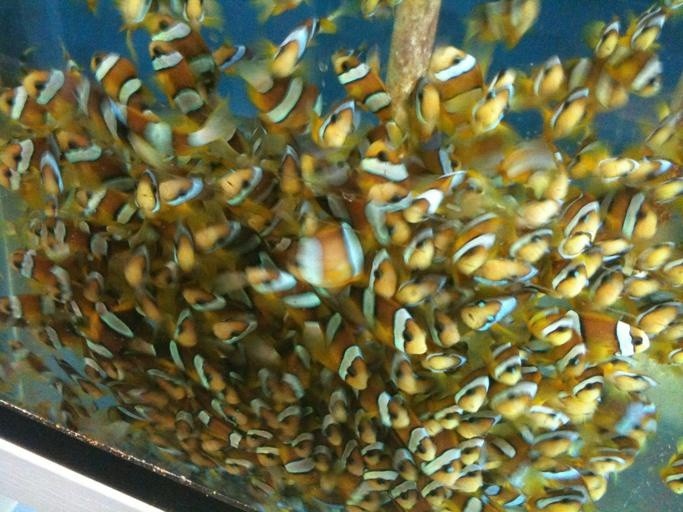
1;0;682;512
